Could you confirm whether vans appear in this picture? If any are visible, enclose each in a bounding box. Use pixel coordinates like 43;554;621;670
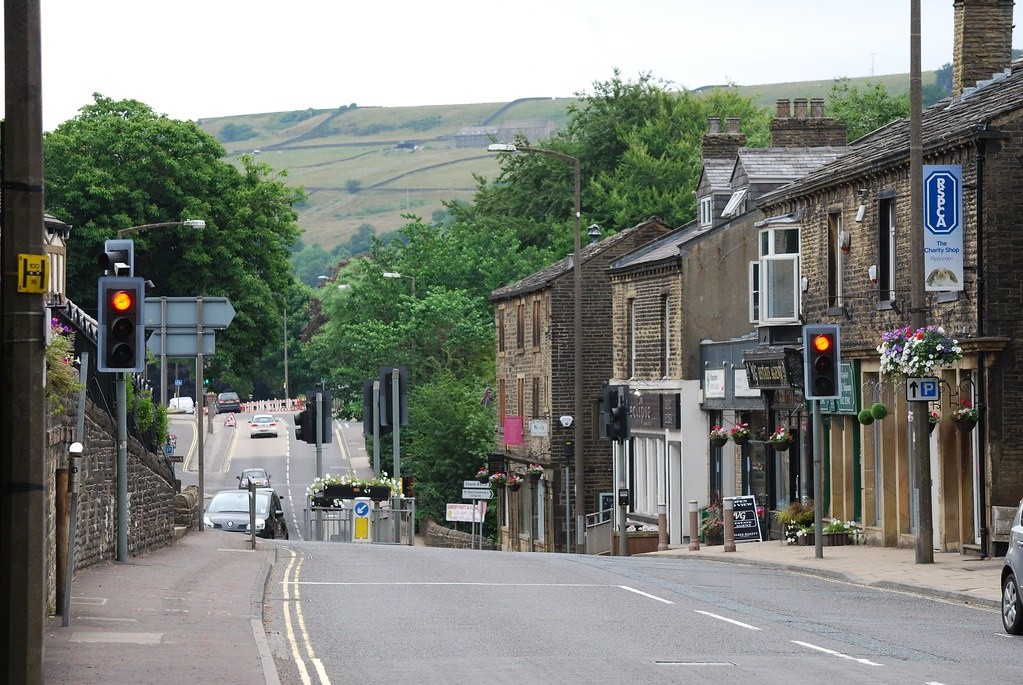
168;396;194;413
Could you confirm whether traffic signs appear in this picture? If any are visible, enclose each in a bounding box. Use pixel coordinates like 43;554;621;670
905;376;940;401
462;480;494;500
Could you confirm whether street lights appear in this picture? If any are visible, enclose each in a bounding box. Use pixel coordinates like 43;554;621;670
115;220;208;562
384;272;418;389
487;142;587;554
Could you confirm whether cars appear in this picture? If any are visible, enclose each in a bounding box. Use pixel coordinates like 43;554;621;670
999;498;1023;634
249;413;279;438
201;488;289;540
236;468;272;488
217;392;242;413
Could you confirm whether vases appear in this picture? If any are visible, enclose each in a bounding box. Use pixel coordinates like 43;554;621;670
704;529;722;546
798;533;815;545
495;482;505;487
734;437;749;444
928;423;936;434
774;442;792;451
509;484;520;492
479;477;489;483
711;439;727;446
821;532;848;545
955;420;977;432
531;474;541;480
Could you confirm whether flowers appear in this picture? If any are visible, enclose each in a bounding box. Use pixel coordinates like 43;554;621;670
876;325;964;388
927;410;940;423
709;425;728;441
699;496;862;544
947;400;981;423
474;467;524;486
529;464;544;476
769;425;795;443
731;423;756;438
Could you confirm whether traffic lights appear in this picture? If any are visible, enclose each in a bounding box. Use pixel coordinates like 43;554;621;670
609;406;630;440
98;278;144;373
293;410;316;444
103;240;134;281
805;323;841;400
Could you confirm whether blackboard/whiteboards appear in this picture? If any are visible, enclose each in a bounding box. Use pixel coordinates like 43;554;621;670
724;495;763;543
